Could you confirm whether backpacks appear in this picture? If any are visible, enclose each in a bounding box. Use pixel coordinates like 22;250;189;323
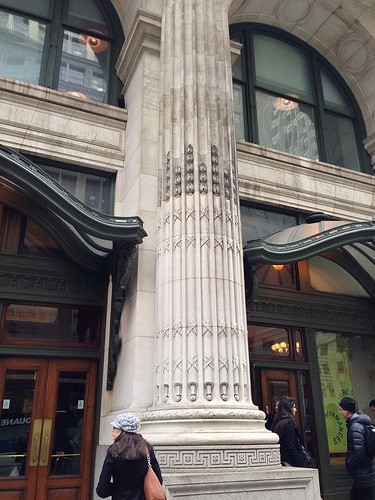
357;422;374;459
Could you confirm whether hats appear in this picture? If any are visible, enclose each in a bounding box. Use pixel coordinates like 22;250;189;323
111;413;140;432
338;397;357;413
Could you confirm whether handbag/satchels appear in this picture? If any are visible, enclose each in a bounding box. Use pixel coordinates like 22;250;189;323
143;445;167;500
295;436;317;467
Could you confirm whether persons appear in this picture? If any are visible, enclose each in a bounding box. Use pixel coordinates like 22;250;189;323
271;396;308;468
339;397;375;500
96;413;163;500
265;397;279;431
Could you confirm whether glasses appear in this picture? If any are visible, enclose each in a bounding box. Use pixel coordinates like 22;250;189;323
113;426;123;430
